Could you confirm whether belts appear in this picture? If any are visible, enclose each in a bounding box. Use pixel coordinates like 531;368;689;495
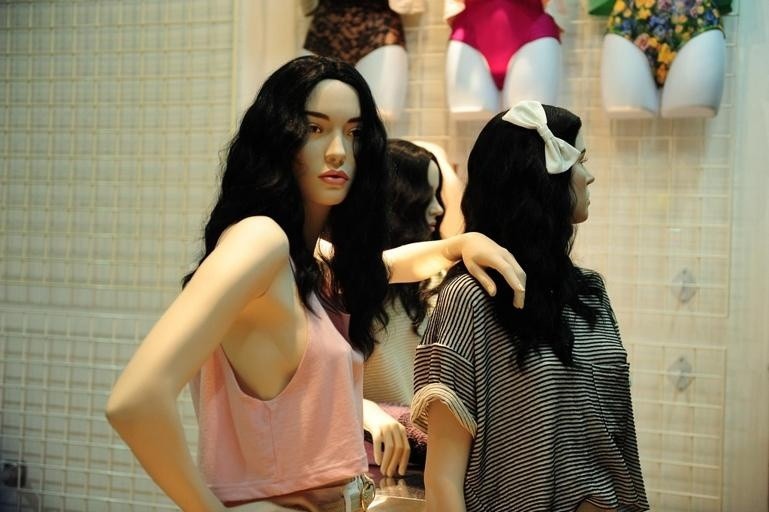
324;474;376;512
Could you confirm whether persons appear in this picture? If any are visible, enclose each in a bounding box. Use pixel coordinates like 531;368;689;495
102;56;529;512
302;0;425;121
586;1;731;120
325;137;461;512
443;1;563;116
410;101;649;511
